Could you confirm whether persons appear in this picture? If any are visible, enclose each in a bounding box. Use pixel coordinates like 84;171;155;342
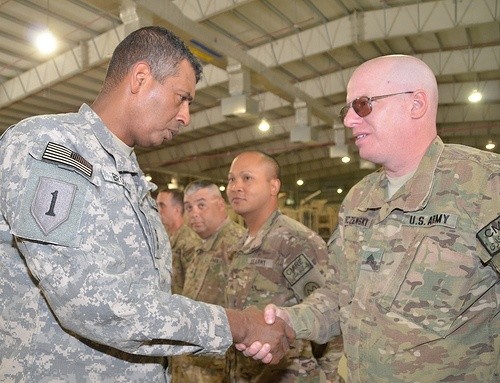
154;185;205;297
231;52;500;383
1;26;297;383
220;150;344;383
162;178;245;383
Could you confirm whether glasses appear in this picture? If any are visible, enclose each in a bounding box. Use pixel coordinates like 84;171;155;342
339;91;414;124
